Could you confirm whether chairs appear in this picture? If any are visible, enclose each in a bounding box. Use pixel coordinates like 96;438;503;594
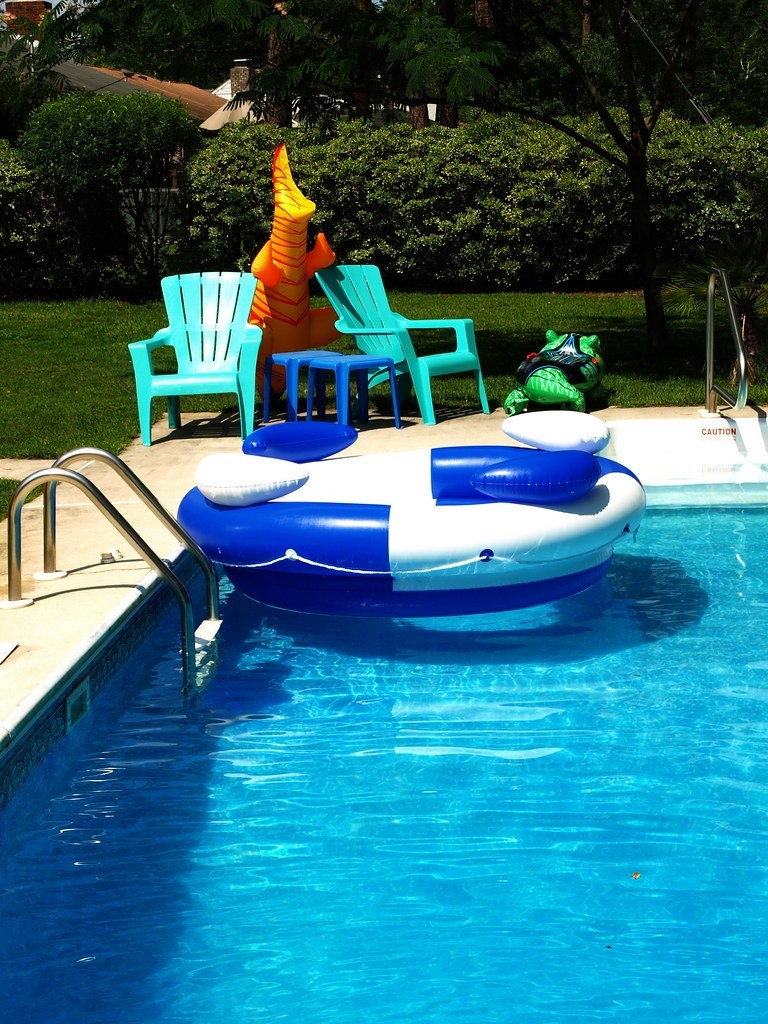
127;271;264;447
315;264;491;427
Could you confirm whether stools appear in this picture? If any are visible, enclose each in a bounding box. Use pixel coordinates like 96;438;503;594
262;349;343;424
307;353;404;430
